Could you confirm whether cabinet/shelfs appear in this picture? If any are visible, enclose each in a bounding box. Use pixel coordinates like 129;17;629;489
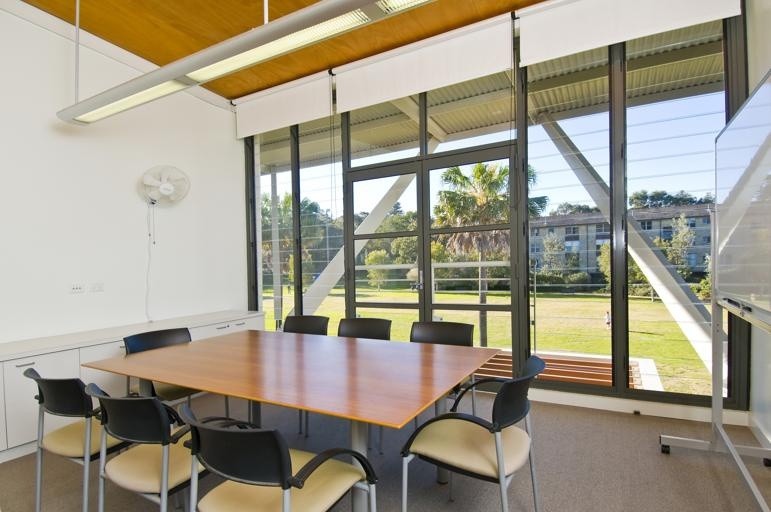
0;309;266;465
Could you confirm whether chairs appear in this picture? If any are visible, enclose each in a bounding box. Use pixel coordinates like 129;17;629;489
378;322;477;454
280;313;331;435
177;402;378;511
399;355;545;512
23;365;144;511
306;317;393;449
83;380;247;511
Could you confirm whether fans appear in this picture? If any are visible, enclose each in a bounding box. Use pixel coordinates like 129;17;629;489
140;165;191;207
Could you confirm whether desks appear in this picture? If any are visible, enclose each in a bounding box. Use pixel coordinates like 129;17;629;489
82;326;504;512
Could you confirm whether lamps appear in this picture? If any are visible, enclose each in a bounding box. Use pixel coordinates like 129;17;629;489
57;0;437;125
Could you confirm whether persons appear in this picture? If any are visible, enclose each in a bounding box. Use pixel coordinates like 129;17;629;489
606;311;610;329
288;286;291;295
